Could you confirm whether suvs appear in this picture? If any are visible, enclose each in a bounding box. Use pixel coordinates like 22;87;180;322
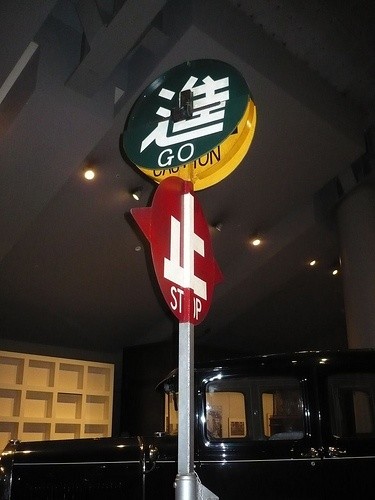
1;348;374;500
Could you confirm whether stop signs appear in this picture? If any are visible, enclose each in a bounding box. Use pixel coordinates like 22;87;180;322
130;176;224;326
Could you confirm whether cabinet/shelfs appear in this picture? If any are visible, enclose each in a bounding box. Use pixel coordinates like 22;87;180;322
0;347;115;456
